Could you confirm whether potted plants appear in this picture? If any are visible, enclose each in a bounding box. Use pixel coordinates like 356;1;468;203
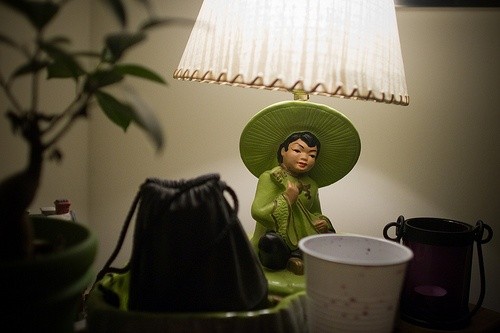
0;0;200;333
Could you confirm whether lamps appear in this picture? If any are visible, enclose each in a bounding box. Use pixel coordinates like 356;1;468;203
173;0;409;296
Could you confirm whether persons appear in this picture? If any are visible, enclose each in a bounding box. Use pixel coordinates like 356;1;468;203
239;100;361;275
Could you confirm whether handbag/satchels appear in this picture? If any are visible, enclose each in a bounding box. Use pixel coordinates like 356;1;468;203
94;172;270;315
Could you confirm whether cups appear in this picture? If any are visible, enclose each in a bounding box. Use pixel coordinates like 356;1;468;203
383;215;494;328
297;234;414;333
